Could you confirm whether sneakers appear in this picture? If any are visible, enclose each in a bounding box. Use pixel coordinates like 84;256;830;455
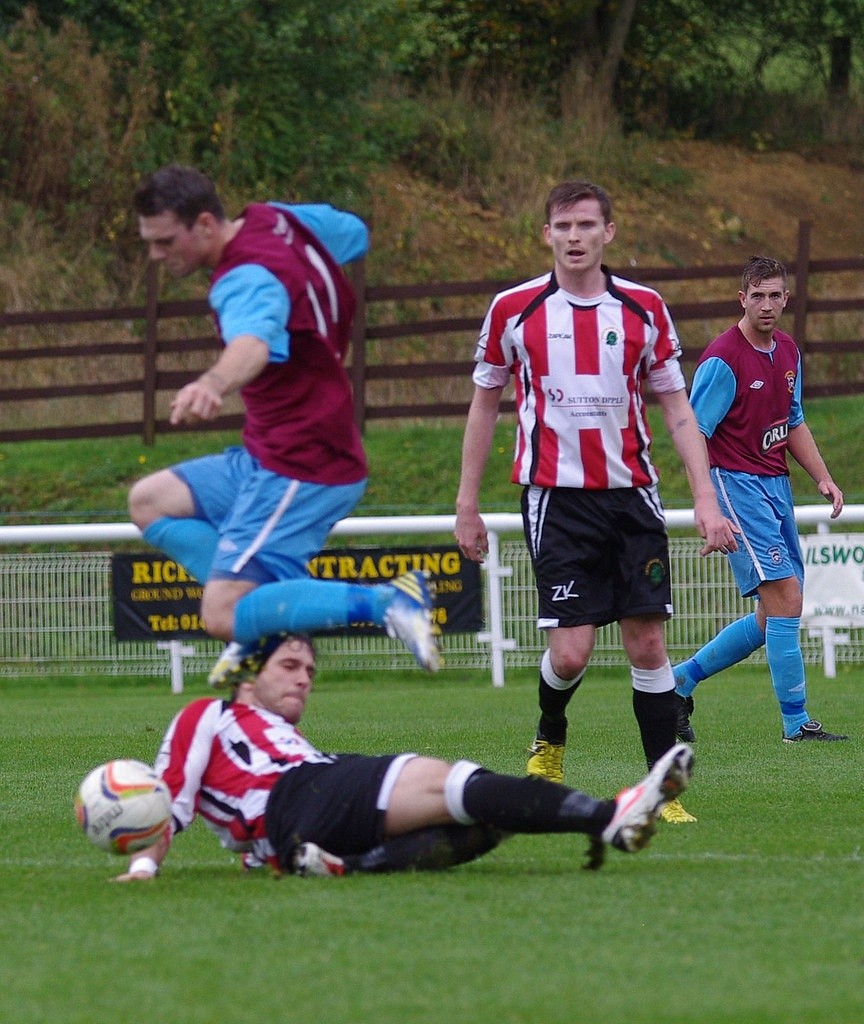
674;690;696;742
526;712;568;783
381;571;439;675
600;742;695;853
654;795;698;823
206;627;286;689
782;718;848;746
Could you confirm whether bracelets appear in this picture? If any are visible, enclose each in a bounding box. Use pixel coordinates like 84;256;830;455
129;857;158;874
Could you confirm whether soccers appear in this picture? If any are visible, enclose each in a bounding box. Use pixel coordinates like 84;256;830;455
73;756;174;856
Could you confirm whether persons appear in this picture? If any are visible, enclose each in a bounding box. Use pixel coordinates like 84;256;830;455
671;255;848;745
128;166;444;689
454;182;740;823
110;633;695;883
